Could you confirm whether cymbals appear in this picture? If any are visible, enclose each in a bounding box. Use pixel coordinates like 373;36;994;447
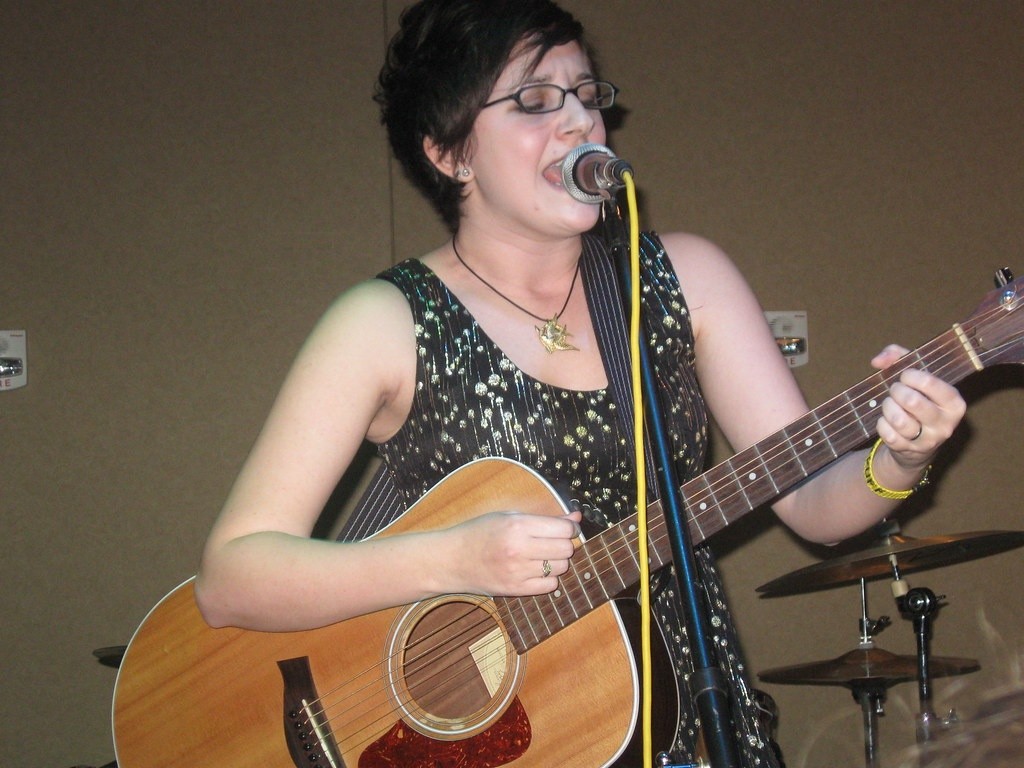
754;529;1024;597
756;647;986;687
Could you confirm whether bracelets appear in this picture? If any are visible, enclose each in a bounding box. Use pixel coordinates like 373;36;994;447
863;437;932;501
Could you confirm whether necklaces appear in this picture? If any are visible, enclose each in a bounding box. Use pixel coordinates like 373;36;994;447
454;235;585;358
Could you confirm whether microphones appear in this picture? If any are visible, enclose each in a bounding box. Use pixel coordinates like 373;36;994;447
559;143;634;203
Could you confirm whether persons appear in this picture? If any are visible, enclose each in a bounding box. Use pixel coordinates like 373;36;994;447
191;0;969;768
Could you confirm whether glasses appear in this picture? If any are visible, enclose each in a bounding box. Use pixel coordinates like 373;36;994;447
483;81;622;115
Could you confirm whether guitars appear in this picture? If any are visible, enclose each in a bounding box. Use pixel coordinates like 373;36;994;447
109;257;1024;768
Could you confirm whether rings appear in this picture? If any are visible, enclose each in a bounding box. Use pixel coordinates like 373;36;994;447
542;560;552;577
910;420;922;440
